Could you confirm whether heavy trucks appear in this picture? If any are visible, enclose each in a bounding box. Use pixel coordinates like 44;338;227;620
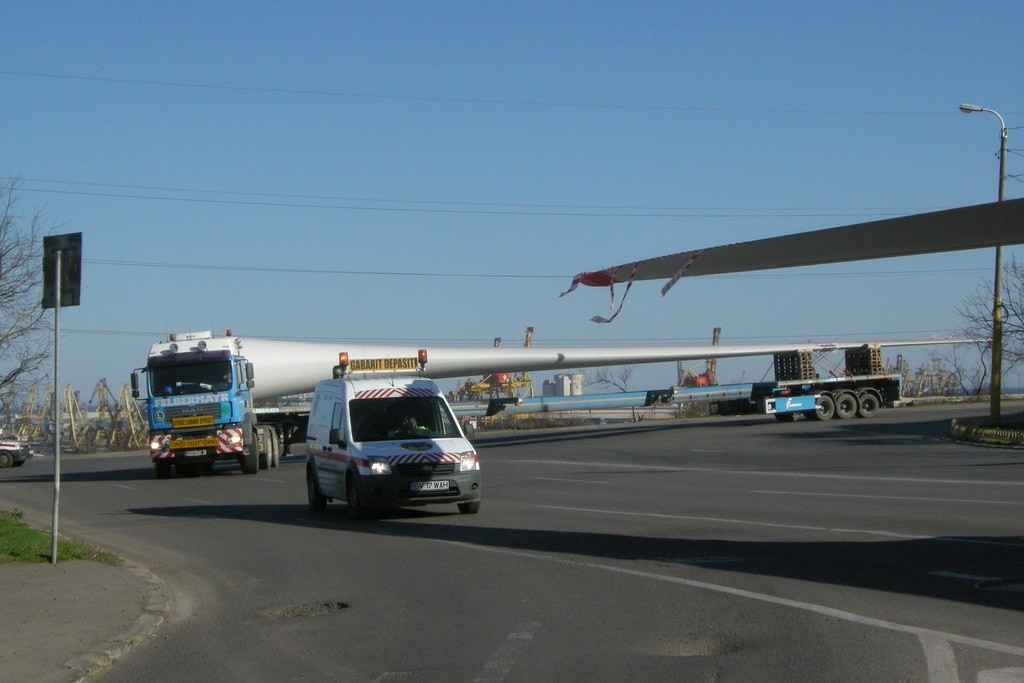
130;328;992;478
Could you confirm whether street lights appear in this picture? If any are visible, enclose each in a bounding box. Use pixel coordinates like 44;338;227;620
960;103;1009;423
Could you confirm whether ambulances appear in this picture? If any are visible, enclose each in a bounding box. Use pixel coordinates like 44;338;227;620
304;350;482;524
